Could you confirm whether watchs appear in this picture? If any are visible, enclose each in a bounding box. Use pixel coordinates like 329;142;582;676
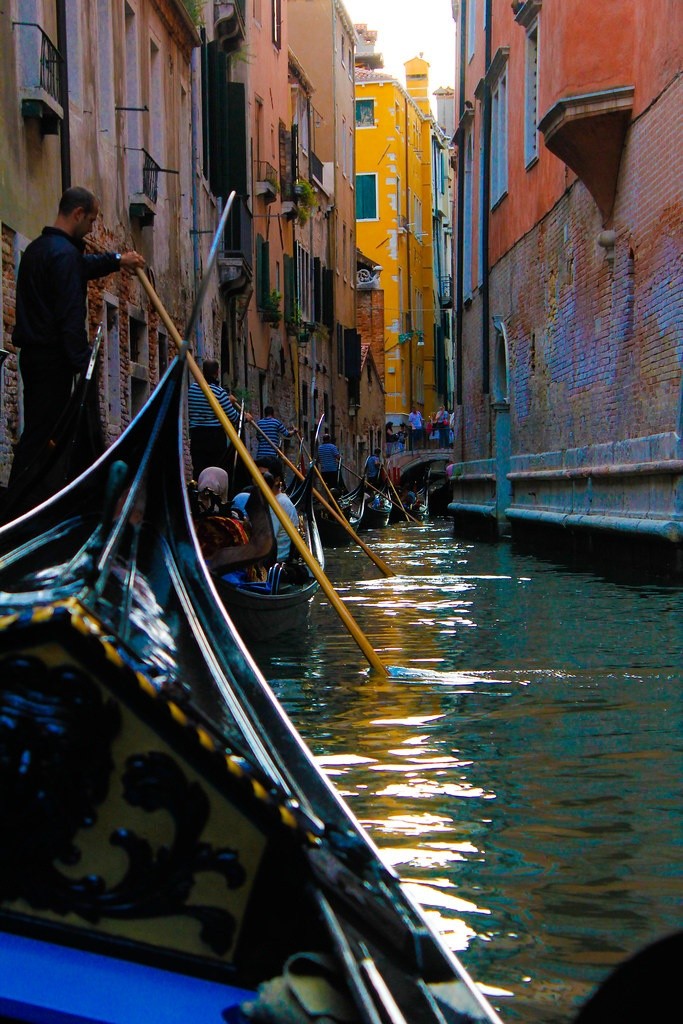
114;252;122;270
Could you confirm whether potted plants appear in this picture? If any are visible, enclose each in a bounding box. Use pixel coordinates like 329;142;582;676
151;0;208;65
284;296;310;347
293;179;312;195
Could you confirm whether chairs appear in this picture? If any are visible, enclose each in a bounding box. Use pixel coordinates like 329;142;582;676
187;480;267;583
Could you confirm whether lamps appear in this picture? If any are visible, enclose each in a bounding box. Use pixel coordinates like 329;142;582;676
408;331;424;346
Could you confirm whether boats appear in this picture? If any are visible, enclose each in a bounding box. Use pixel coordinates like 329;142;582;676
0;189;501;1023
186;412;326;640
361;461;393;528
313;463;369;549
281;436;304;498
389;468;432;522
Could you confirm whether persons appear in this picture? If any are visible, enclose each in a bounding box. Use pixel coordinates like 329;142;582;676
397;401;455;453
188;358;253;485
366;447;383;486
386;422;398;455
317;434;342;489
251;455;300;562
5;185;147;519
256;405;299;468
